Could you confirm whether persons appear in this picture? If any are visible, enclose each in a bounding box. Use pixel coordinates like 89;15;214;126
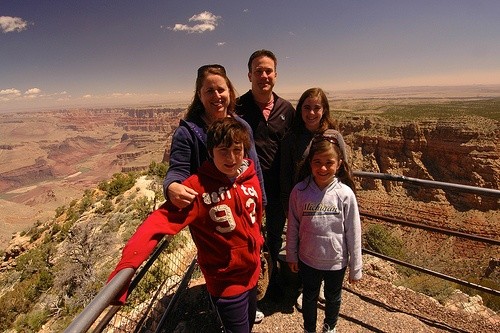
233;49;301;307
104;118;270;333
285;137;362;333
162;68;267;323
280;90;357;312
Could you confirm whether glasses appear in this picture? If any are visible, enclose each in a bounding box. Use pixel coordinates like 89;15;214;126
312;138;339;146
198;64;226;76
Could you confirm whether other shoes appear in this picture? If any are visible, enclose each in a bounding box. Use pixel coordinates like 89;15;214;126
297;293;303;309
255;308;265;322
318;286;326;302
323;323;337;333
